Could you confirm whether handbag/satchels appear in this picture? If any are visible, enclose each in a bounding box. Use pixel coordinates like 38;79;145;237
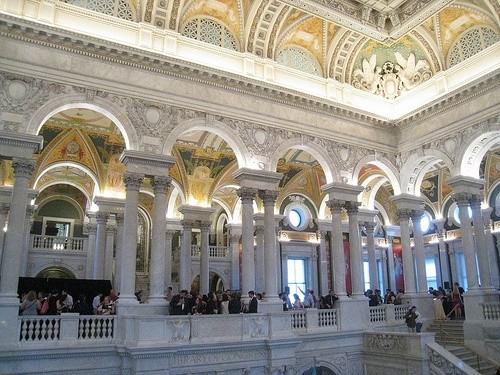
19;310;23;315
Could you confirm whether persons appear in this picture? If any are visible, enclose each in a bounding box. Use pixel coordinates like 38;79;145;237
164;287;266;315
92;288;120;315
279;288;341;311
363;288;405;306
429;281;465;319
19;289;73;315
404;306;423;333
135;290;144;304
